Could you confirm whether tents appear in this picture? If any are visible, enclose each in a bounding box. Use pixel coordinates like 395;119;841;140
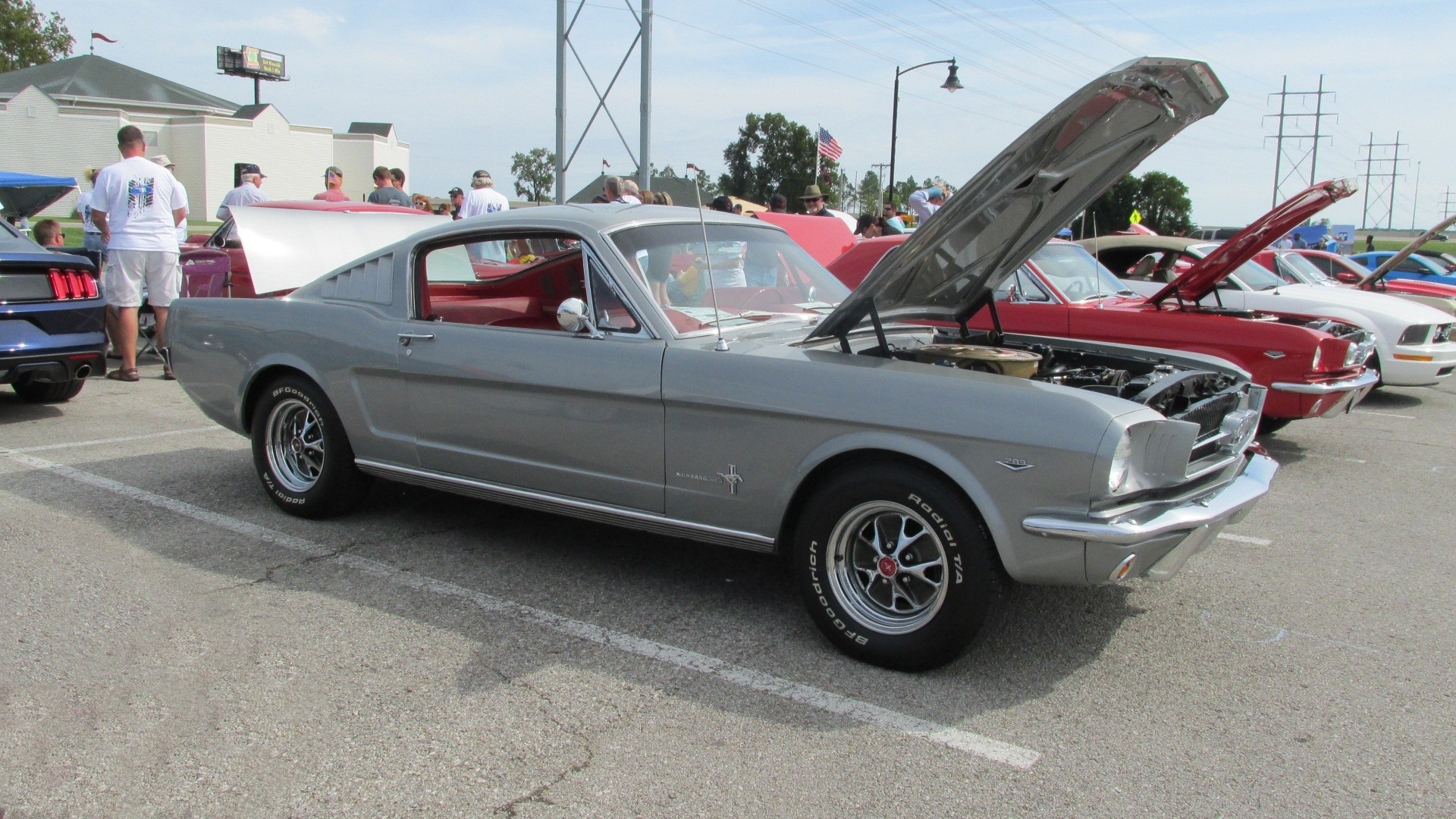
0;171;82;222
1055;227;1073;241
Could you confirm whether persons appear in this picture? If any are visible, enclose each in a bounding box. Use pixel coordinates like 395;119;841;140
1278;233;1307;249
1313;239;1326;249
1366;235;1374;251
313;166;351;201
593;176;673;307
216;165;267;234
76;166;110;276
694;194;786;288
448;187;464;219
150;154;190;244
33;219;63;247
367;166;413;207
439;203;452;215
412;193;432;212
107;278;147;359
853;181;954;238
797;184;834;217
458;170;510;263
89;125;187;381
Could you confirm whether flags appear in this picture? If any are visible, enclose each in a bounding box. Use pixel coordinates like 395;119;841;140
328;171;342;186
819;127;843;161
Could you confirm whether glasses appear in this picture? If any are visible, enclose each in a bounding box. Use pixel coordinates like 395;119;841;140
391;179;399;183
804;198;821;203
439;210;444;213
860;226;869;235
415;202;426;205
58;233;65;238
884;207;892;210
450;193;460;198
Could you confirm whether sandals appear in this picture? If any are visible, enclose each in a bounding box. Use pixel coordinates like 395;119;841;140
163;366;175;379
108;363;140;381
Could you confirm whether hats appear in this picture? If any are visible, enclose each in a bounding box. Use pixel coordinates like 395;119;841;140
471;170;491;187
241;164;266;178
323;166;342;178
796;185;829;201
151;155;176;168
624;180;638;195
449;187;463;196
853;214;874;235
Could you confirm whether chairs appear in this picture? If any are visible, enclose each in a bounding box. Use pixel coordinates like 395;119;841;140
1153;252;1179;283
1128;255;1156;281
137;248;234;366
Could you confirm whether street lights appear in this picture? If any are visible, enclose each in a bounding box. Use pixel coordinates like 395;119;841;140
889;57;965;203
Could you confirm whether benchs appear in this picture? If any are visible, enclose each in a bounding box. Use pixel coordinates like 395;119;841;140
430;296;544;328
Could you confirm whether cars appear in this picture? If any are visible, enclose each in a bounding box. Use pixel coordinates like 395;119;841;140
164;56;1455;677
0;216;108;403
